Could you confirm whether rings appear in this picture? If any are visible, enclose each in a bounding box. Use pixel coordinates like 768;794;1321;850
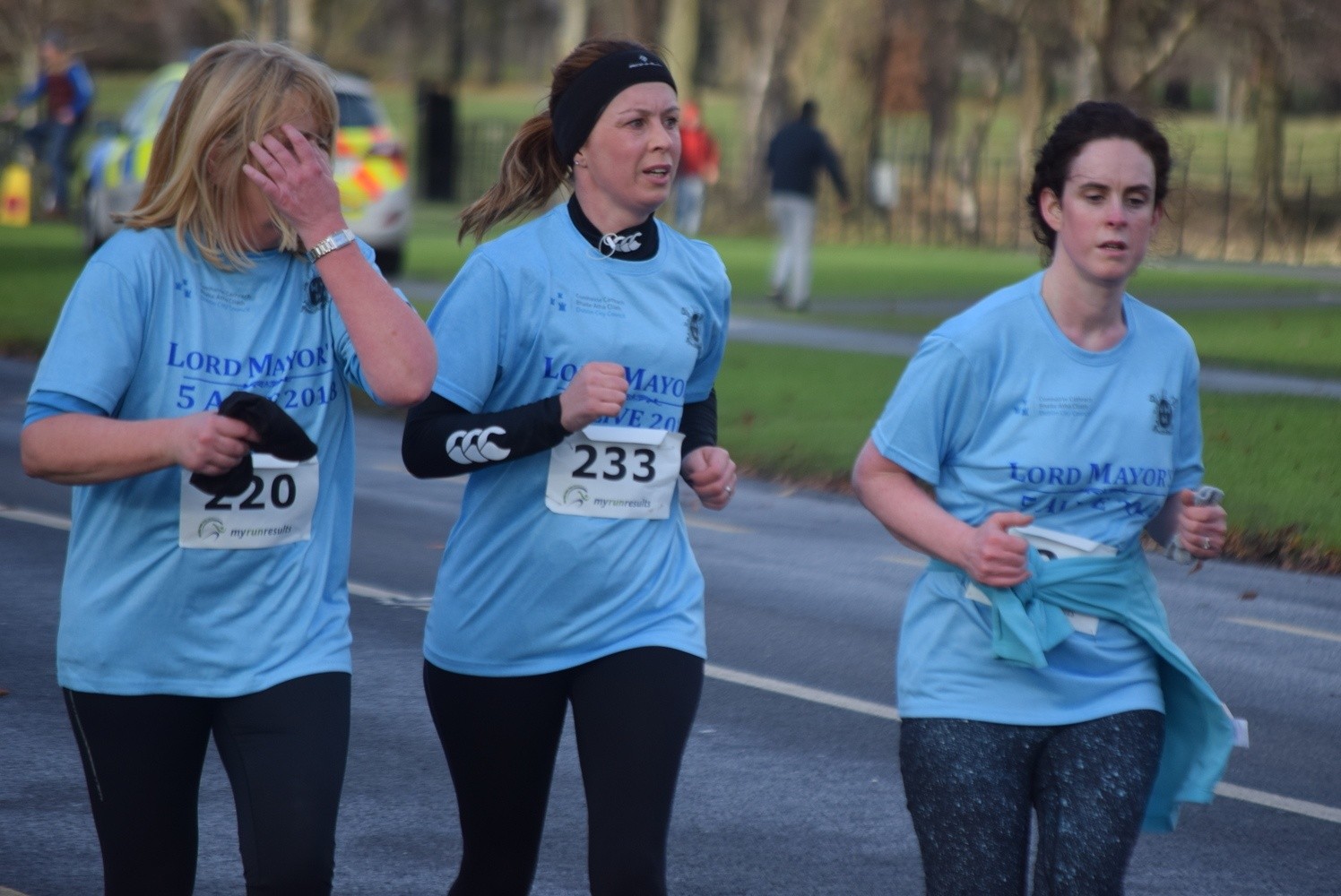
725;486;731;494
1203;537;1211;550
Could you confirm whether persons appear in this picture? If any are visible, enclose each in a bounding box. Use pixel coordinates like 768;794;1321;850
0;30;96;220
402;37;737;896
18;40;439;895
760;97;854;309
669;102;720;237
847;100;1232;896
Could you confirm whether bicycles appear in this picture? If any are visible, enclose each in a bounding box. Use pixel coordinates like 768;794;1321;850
0;116;87;224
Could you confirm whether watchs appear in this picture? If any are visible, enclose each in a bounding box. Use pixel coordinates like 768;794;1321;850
308;229;355;264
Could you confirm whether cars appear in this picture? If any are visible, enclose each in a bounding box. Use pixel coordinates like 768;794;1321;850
82;46;409;277
